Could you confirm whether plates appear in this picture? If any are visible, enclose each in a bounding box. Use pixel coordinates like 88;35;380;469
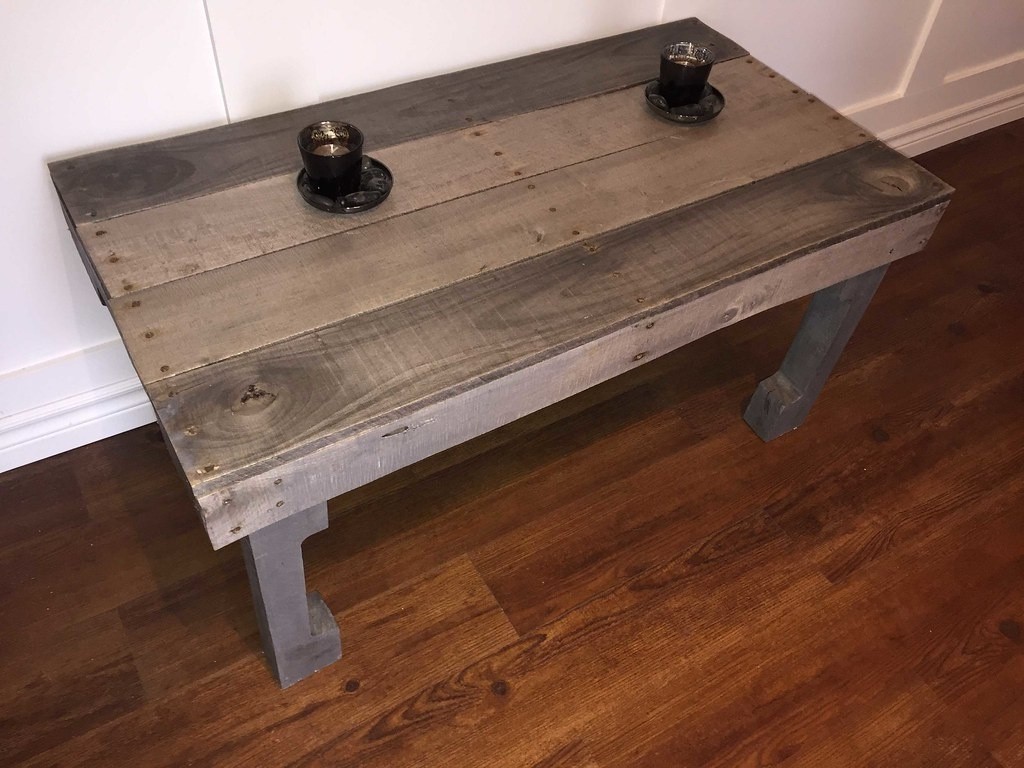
644;78;725;122
296;155;394;214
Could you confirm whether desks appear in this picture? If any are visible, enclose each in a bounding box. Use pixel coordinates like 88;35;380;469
48;16;956;688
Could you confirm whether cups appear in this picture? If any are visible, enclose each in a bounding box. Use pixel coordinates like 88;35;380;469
660;42;716;106
296;119;364;190
